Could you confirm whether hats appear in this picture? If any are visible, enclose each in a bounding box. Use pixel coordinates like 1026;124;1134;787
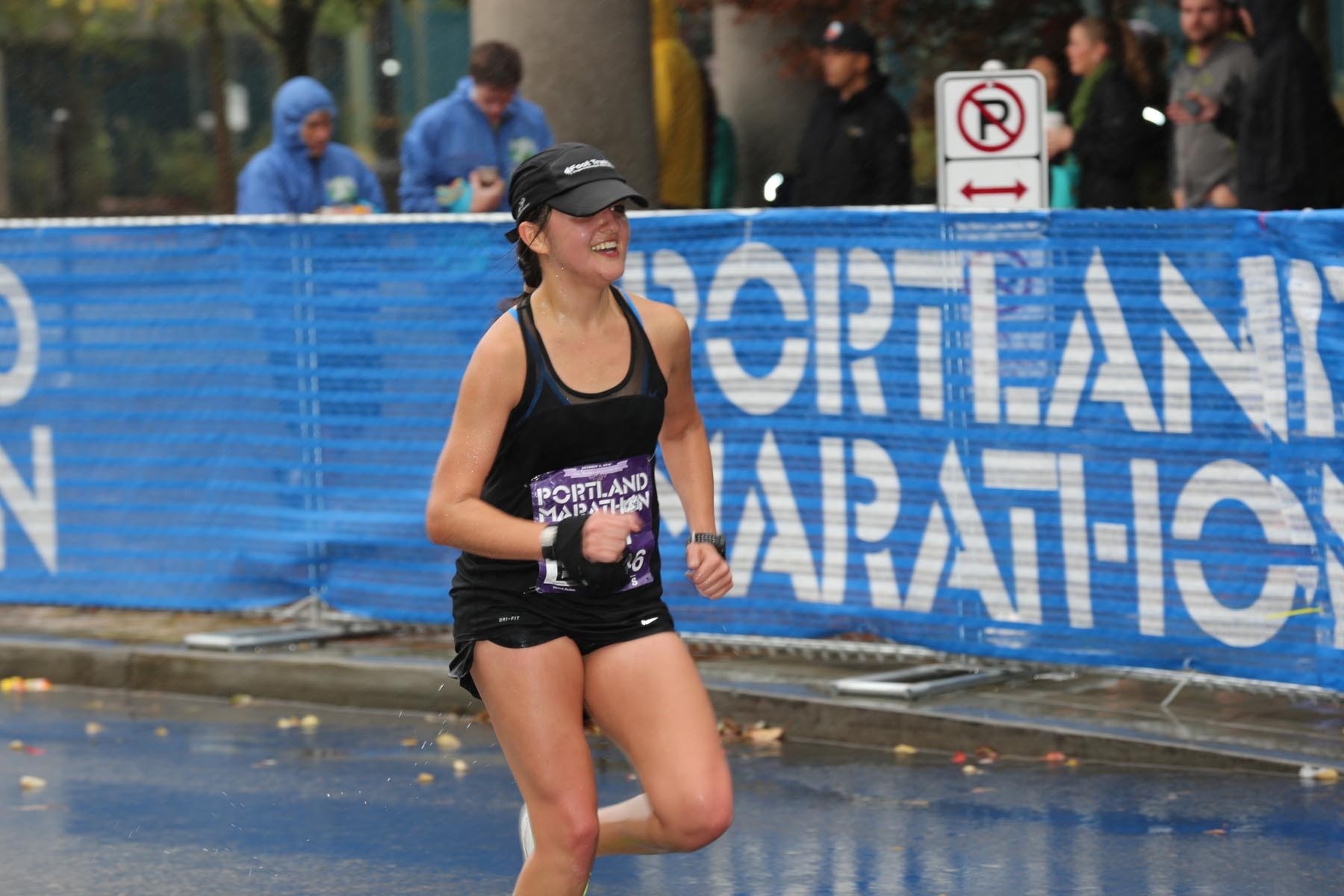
811;19;874;54
505;141;648;243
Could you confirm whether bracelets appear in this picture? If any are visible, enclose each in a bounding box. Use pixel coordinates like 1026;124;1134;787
540;520;556;560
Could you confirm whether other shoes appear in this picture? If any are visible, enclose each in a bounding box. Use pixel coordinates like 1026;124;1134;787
521;802;588;895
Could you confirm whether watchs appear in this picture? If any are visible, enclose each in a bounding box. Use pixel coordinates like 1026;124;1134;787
687;532;725;554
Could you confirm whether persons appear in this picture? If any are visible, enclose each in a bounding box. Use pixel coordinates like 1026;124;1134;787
765;17;913;206
1023;0;1297;209
395;40;553;213
233;76;386;214
425;142;733;896
